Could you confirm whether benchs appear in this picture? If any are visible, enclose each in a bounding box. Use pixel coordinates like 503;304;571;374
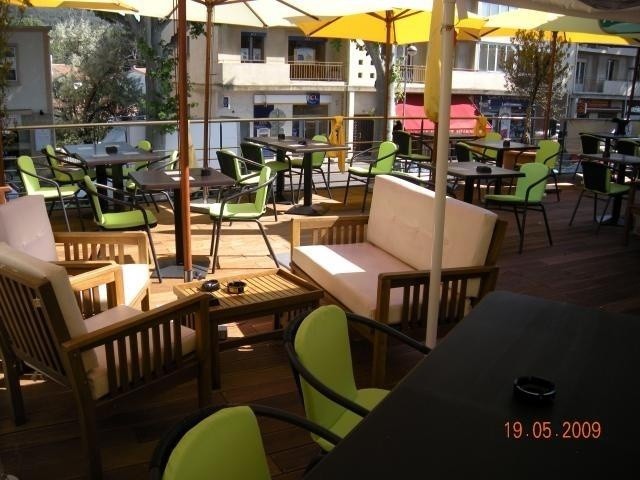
287;174;507;389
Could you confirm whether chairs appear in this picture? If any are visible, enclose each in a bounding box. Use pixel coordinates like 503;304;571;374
0;241;212;475
9;132;639;274
282;305;432;472
0;194;152;320
149;401;342;480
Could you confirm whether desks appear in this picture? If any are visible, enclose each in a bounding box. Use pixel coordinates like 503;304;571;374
300;288;639;480
172;267;322;388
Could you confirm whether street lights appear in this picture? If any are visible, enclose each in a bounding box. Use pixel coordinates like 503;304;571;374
400;44;417;130
